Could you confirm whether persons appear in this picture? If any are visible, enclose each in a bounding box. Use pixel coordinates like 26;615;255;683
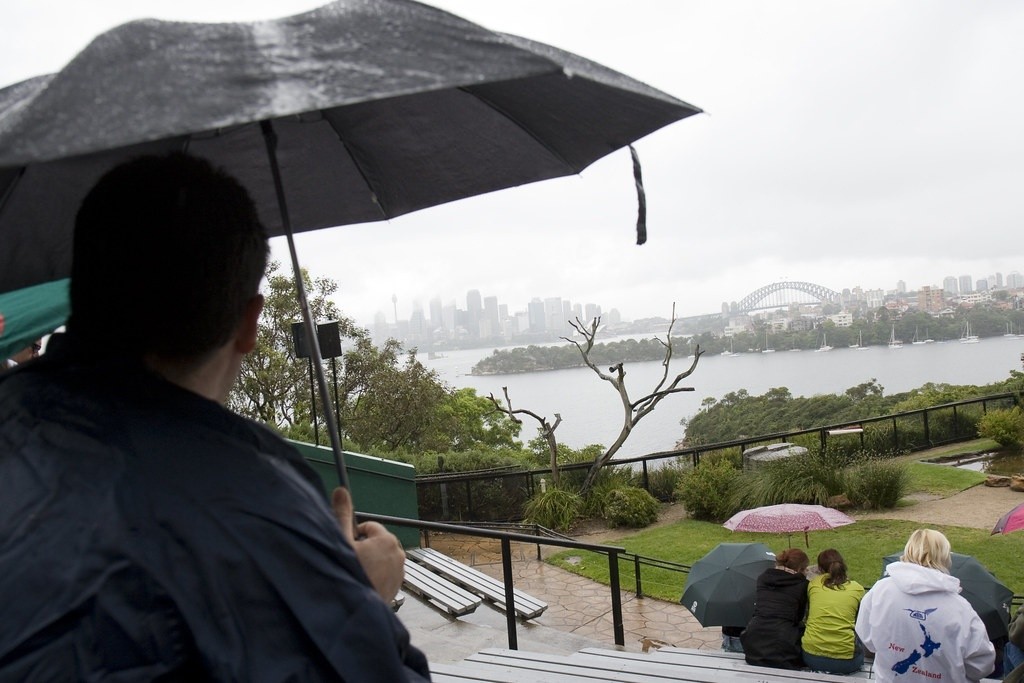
5;328;42;370
1001;604;1024;683
801;548;865;675
855;528;995;683
0;152;434;683
720;625;746;654
744;548;810;672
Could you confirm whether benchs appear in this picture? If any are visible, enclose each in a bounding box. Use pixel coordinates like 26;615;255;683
389;546;551;619
428;646;879;683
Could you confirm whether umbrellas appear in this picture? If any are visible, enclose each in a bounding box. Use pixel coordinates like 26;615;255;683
990;503;1024;536
680;543;779;628
0;0;710;538
721;502;856;550
882;549;1015;646
